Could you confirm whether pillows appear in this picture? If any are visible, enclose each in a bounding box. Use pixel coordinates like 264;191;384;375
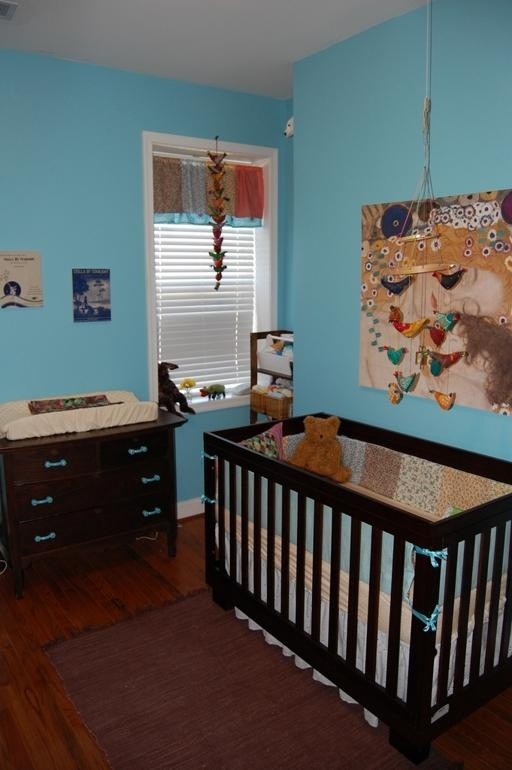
237;423;284;461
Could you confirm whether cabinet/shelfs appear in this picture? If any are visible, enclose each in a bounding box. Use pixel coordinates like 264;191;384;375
0;407;188;598
249;330;294;424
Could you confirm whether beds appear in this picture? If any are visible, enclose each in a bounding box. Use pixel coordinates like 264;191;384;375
203;410;512;766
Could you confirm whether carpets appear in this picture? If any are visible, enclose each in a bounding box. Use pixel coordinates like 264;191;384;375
41;585;465;770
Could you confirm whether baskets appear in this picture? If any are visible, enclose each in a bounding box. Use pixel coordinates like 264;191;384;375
251;390;264;414
262;394;292;420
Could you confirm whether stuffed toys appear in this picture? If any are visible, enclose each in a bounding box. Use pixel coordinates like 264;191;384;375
158;362;195;418
288;416;352;483
